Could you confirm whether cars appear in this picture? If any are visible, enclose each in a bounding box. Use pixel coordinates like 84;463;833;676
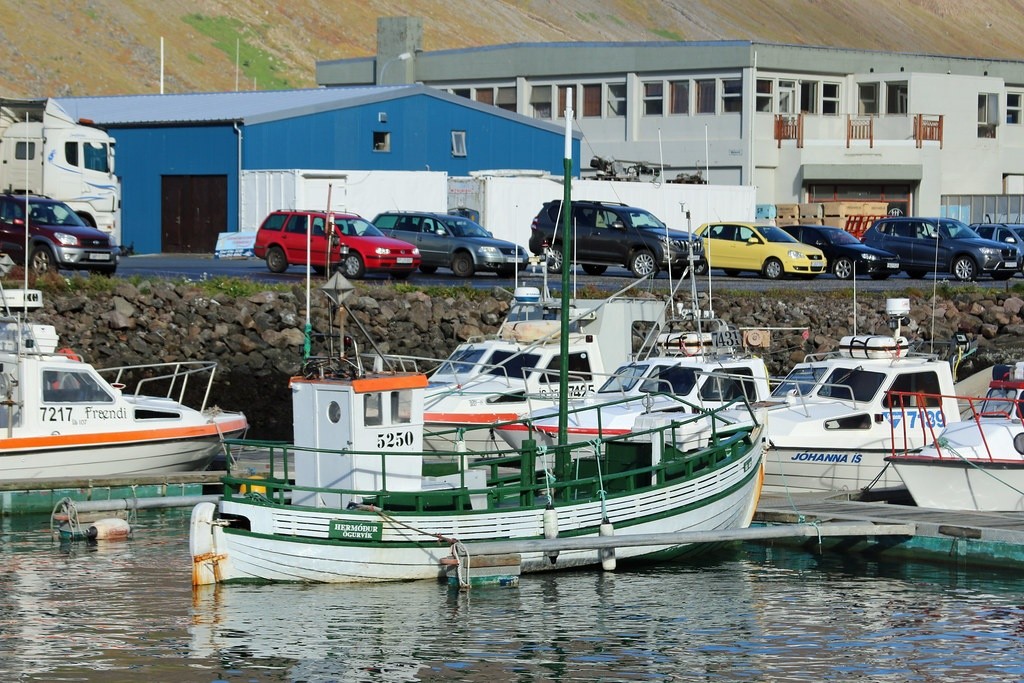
693;221;827;280
0;188;119;279
779;225;902;281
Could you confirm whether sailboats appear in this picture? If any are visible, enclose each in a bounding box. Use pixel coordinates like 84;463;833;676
187;88;771;588
419;123;1023;512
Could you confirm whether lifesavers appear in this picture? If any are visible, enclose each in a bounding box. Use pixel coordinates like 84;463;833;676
57;347;79;363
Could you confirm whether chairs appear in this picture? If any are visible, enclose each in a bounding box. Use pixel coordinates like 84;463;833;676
596;213;608;227
916;224;925;238
424;222;431;232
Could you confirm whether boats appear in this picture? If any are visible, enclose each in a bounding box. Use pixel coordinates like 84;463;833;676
0;283;250;514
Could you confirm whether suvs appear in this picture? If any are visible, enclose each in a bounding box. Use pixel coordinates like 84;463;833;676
953;222;1024;278
529;199;706;280
252;209;422;281
363;211;529;279
860;216;1022;282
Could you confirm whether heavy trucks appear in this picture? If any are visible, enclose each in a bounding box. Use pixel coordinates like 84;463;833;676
0;97;121;244
